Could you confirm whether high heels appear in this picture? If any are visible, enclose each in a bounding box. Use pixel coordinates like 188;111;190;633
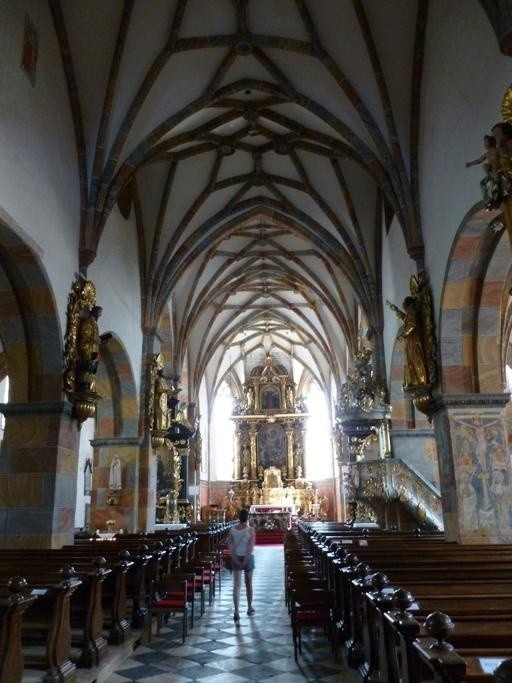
247;608;255;615
233;614;240;623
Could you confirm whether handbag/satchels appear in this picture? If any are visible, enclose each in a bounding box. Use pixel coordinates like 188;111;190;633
222;555;232;570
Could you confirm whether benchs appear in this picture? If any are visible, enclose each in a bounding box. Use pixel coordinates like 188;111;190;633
1;518;242;683
284;521;512;683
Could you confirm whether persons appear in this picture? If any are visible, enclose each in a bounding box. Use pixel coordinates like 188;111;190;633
259;429;285;467
108;453;123;490
344;500;357;524
450;414;512;532
77;303;104;392
384;295;428;388
247;387;255;404
464;119;511;195
154;374;175;429
285;386;293;408
227;508;256;622
